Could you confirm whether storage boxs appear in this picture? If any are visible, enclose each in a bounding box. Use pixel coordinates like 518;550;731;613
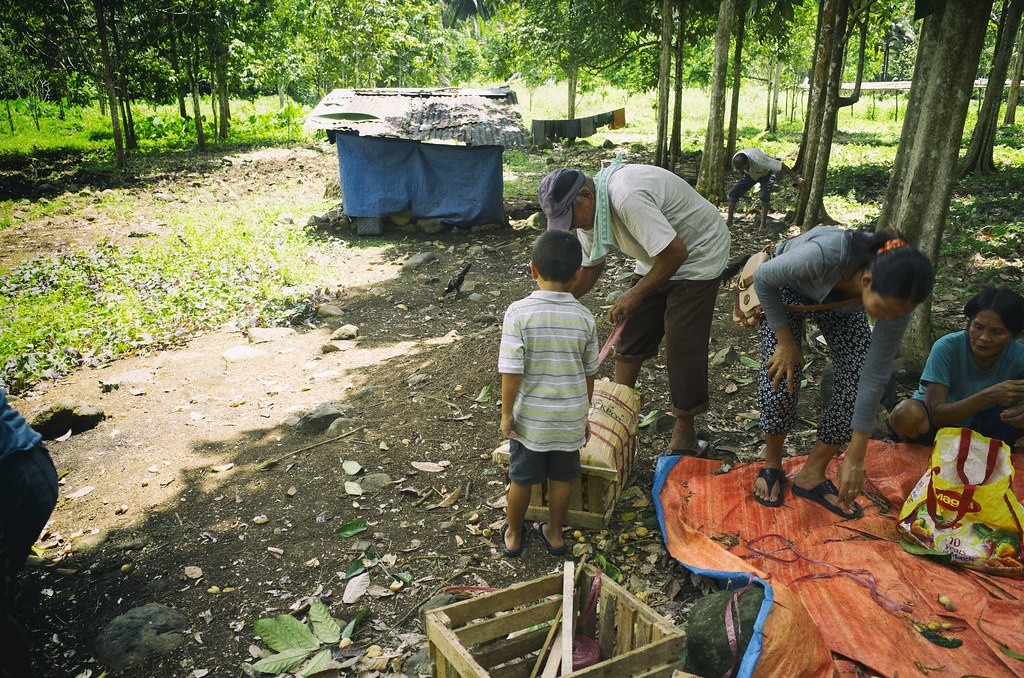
424;564;686;678
491;378;639;531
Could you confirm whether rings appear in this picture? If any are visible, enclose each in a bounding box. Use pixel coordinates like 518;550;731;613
848;490;853;493
853;489;858;492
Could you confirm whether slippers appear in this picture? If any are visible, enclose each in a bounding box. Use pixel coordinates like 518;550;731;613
501;523;524;556
793;479;861;519
535;521;567;555
752;468;785;507
664;439;709;457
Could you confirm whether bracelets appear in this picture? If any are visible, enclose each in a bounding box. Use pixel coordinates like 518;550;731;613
846;462;865;471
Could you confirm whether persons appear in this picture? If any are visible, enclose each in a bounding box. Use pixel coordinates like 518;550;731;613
0;388;59;642
538;163;730;458
725;148;803;234
498;229;598;555
889;286;1024;443
752;225;933;518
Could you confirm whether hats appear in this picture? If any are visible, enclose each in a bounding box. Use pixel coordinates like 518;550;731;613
538;168;587;232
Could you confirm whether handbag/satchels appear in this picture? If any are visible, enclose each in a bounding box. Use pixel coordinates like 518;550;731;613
896;427;1024;577
732;244;773;328
